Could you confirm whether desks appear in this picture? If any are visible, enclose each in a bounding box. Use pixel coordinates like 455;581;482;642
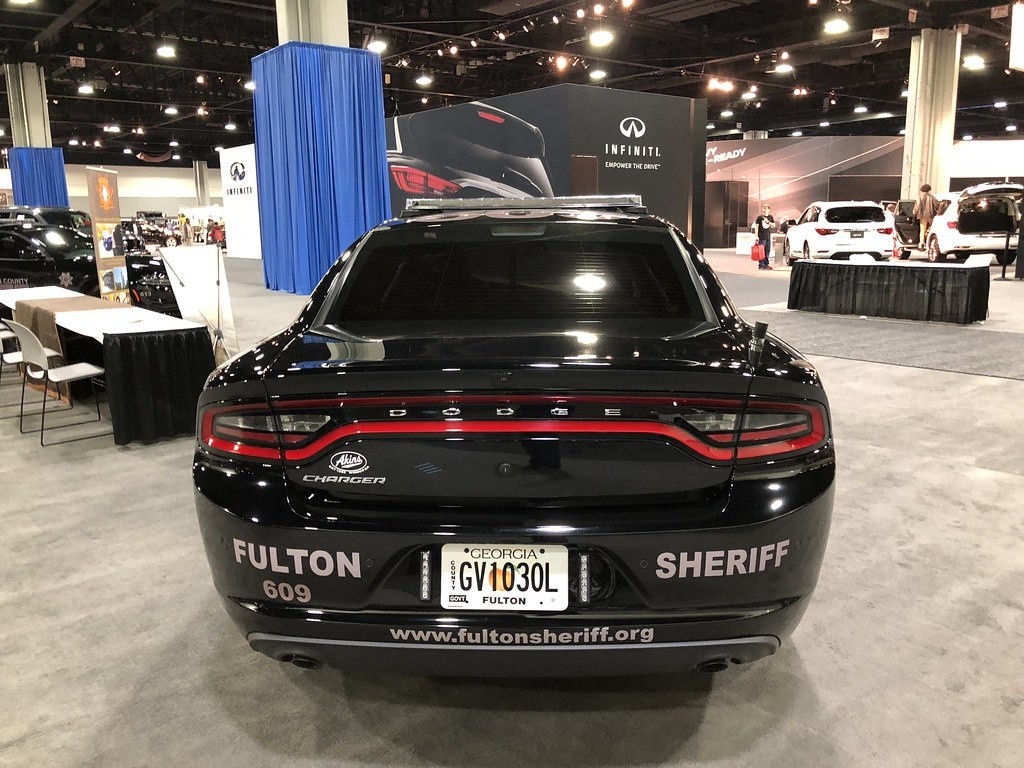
0;283;215;449
785;255;993;330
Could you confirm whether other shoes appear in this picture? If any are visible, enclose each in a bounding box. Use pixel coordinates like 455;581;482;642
761;266;773;270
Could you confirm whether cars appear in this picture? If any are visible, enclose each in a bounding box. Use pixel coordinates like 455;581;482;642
894;178;1024;266
0;205;150;256
783;198;894;266
190;189;839;683
1;220;185;318
121;217;181;248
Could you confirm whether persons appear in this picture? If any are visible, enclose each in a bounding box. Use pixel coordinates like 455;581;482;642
886;204;901;258
149;212;226;247
754;203;777;271
912;183;940;249
126;216;144;237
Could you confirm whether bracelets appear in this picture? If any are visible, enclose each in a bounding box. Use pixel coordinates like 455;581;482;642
766;221;770;224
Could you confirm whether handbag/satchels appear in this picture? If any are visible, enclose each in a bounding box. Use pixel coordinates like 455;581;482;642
751;240;765;261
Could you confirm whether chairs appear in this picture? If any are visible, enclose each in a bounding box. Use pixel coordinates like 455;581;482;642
0;346;73;420
0;318;116;445
